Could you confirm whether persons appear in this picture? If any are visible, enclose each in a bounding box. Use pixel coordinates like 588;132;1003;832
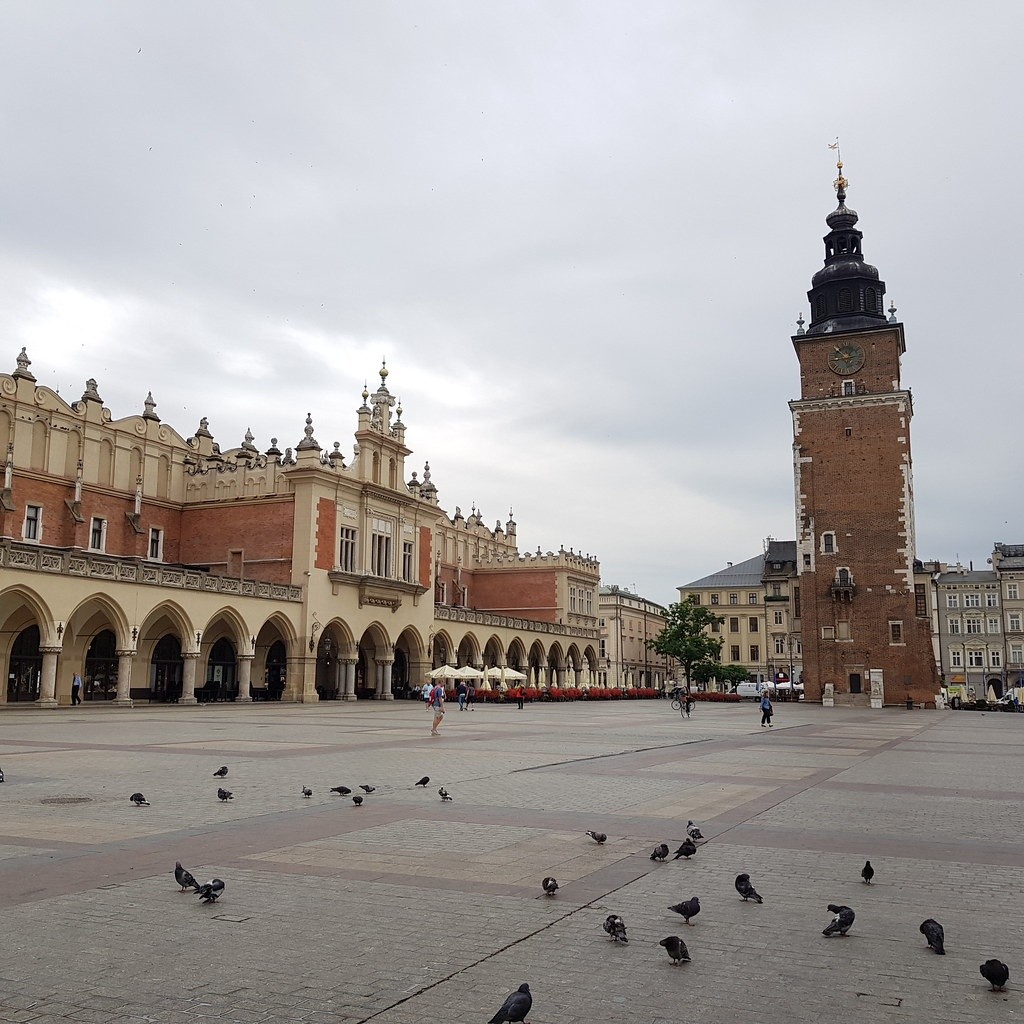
373;404;384;423
422;679;434;712
457;682;467;711
435;680;447;712
516;685;524;709
411;684;426;701
1008;697;1019;712
70;672;82;705
764;689;804;702
430;680;446;735
658;687;684;700
759;690;773;727
681;686;696;713
465;682;475;711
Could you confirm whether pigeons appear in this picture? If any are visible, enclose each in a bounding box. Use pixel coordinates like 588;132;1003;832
649;843;669;862
0;762;235;808
487;982;534;1024
301;785;312;798
687;820;704;842
667;898;700;926
415;776;430;787
920;919;945;955
603;914;629;942
353;796;363;806
585;830;607;845
735;874;763;904
822;905;855;937
438;786;452;802
672;838;697;860
659;936;691;967
541;877;559;897
174;861;225;902
861;862;874;884
358;785;376;794
330;786;351;796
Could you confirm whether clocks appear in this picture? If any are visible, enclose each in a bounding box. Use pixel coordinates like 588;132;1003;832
827;340;866;376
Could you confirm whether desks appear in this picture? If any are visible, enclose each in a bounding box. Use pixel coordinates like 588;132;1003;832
196;688;212;703
254;688;267;702
225;689;237;702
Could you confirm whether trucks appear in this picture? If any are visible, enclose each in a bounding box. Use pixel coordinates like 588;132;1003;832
726;685;737;696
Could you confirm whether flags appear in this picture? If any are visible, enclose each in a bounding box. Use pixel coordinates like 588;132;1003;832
828;142;838;150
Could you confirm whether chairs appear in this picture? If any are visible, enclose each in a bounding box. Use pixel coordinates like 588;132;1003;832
444;687;655;703
164;681;254;703
692;692;742;703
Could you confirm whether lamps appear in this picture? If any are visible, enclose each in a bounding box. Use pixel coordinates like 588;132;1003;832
57;621;63;640
251;635;256;650
392;643;395;653
605;652;611;670
196;631;201;646
567;654;571;675
355;641;359;653
309;612;326;654
427;624;436;659
131;627;138;641
455;650;458;658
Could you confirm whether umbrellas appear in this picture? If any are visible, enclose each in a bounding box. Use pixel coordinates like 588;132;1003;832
640;674;717;693
960;684;1024;705
425;664;527;690
529;667;634;690
761;681;804;694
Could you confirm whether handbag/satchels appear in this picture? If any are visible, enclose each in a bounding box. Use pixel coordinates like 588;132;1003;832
769;703;774;716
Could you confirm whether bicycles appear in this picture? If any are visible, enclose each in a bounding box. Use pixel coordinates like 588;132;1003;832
670;685;696;719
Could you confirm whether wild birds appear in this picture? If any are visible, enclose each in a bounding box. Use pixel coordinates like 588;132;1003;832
979;959;1010;993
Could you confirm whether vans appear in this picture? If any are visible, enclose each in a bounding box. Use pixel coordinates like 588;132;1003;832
737;682;769;702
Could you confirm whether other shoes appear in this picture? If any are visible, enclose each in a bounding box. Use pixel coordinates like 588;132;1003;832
769;724;773;728
761;723;766;728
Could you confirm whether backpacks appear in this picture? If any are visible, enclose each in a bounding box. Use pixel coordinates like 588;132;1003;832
428;687;440;706
